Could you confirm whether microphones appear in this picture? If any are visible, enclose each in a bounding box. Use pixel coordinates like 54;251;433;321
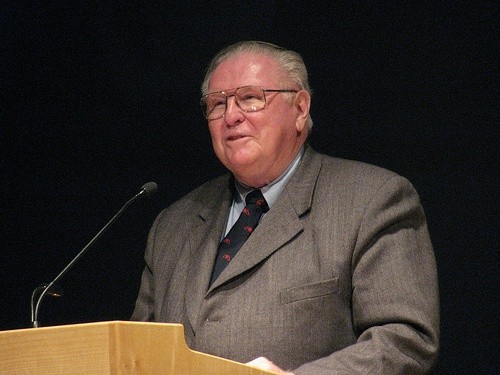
30;181;159;329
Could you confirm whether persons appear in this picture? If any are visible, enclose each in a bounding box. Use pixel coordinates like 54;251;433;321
129;41;440;375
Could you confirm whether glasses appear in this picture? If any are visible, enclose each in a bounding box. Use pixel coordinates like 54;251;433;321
200;86;297;121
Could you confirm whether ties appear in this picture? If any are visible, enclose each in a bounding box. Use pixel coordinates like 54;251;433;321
209;188;269;287
